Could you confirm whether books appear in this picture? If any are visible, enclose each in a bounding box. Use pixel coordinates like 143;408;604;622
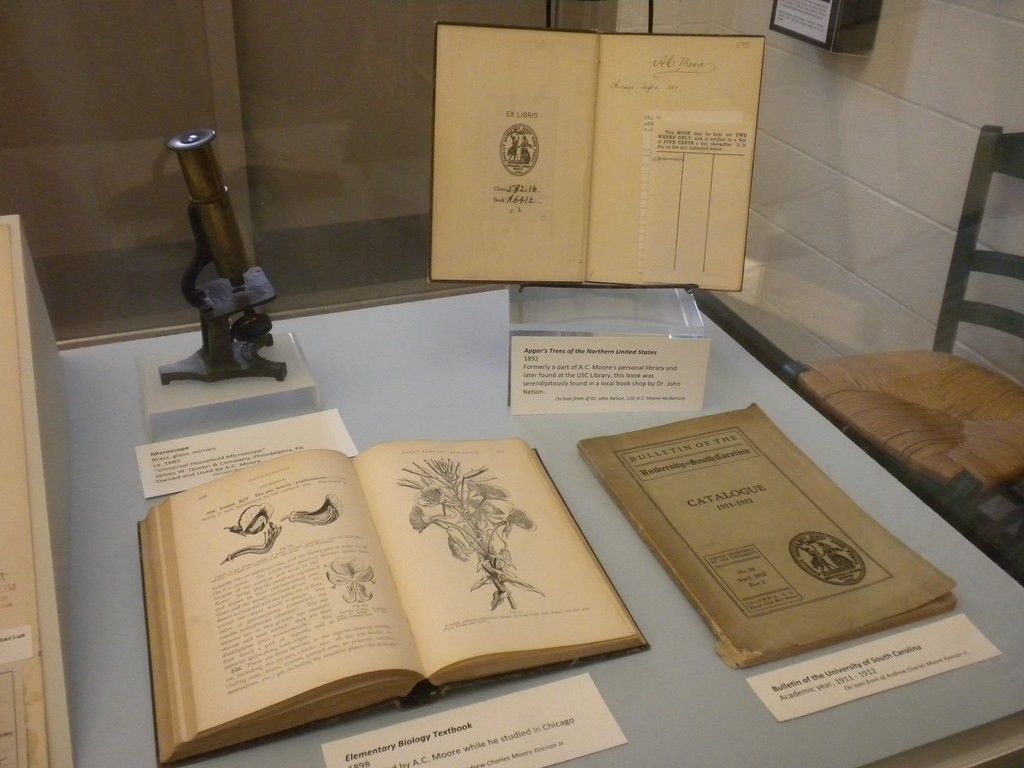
431;22;765;292
138;437;650;768
578;402;956;668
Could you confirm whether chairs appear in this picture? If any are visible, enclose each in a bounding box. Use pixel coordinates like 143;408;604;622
782;124;1024;582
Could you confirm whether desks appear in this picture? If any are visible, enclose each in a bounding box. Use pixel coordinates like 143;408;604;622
48;276;1023;768
